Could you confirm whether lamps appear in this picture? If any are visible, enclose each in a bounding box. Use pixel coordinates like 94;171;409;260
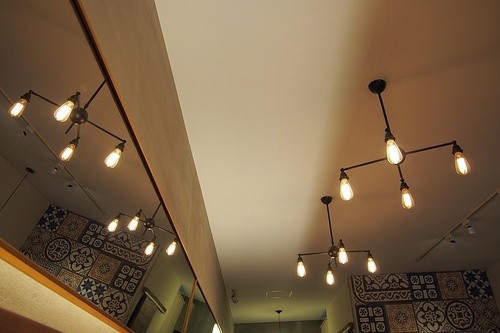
337;79;472;210
295;197;379;286
108;202;178;257
447;233;456;248
462;220;476;235
7;79;127;169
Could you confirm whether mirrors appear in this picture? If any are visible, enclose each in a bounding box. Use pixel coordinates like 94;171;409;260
0;1;223;333
